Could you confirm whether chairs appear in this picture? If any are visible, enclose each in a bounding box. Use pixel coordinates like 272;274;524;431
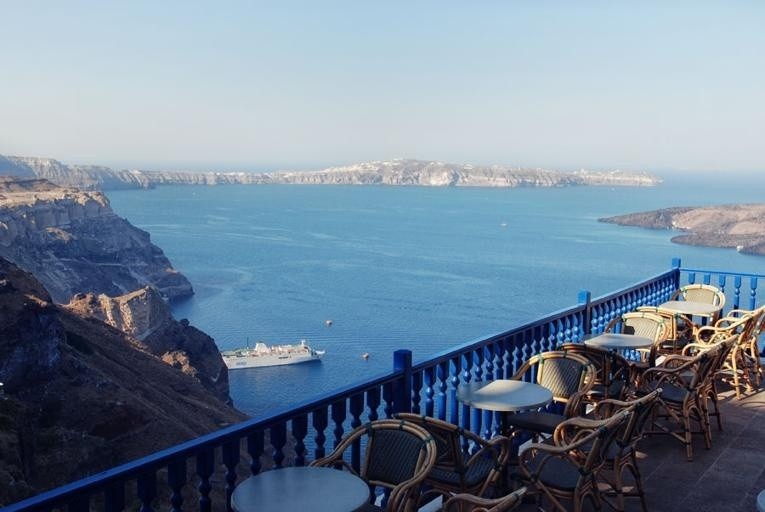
454;351;664;510
557;311;738;463
622;284;765;401
392;413;527;511
311;419;437;510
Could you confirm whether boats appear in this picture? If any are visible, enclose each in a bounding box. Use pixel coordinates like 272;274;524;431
220;336;326;370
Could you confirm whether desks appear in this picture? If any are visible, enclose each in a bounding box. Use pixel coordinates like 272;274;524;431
227;465;373;511
